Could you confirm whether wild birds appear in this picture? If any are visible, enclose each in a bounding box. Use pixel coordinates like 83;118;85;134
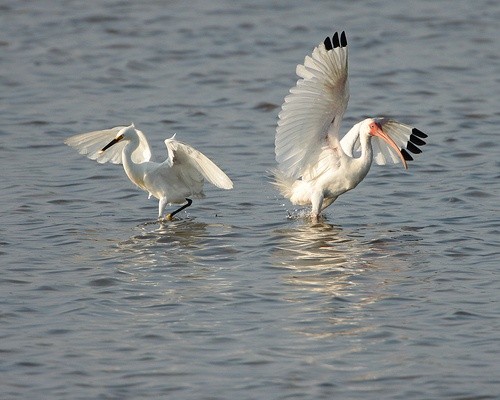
63;121;235;221
262;30;428;218
